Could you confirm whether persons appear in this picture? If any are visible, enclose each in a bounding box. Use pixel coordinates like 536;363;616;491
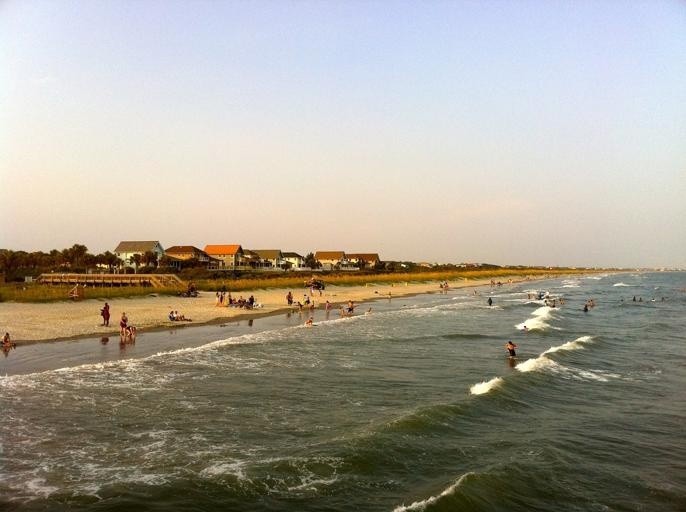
2;346;10;358
125;326;136;336
0;331;10;344
101;336;110;345
119;336;127;354
439;279;596;313
173;311;186;321
119;311;128;335
215;279;359;326
127;336;135;346
168;311;181;321
523;325;529;332
505;341;518;358
99;303;110;327
508;358;516;368
